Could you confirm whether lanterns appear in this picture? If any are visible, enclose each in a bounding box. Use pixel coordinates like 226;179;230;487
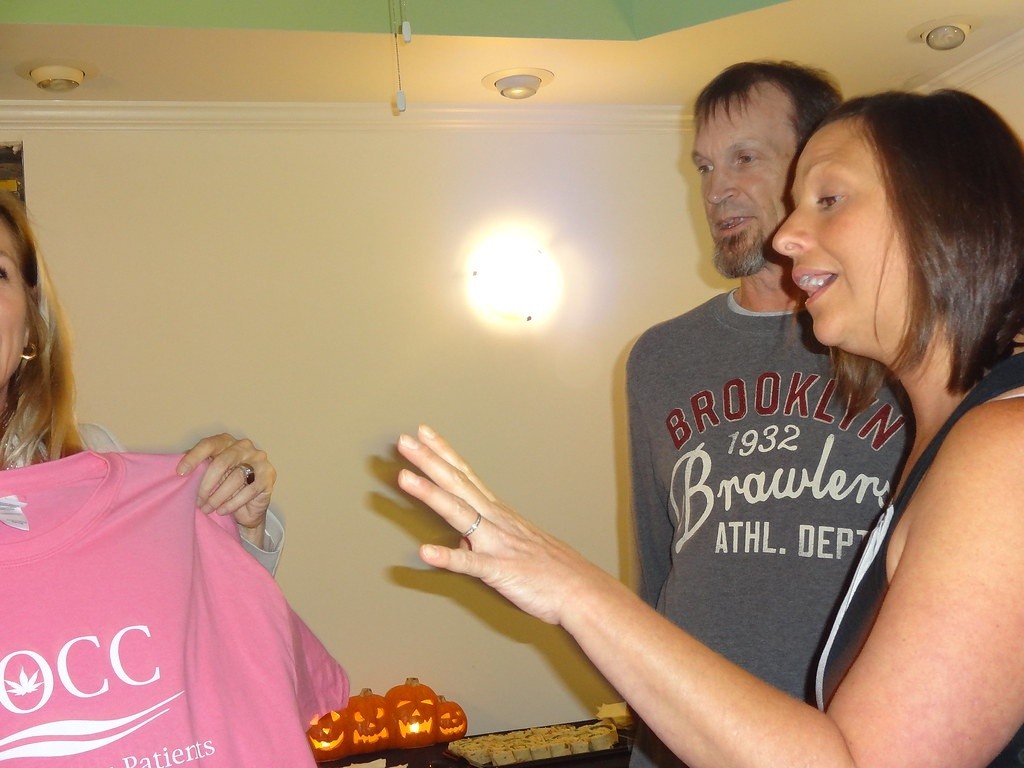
307;675;467;762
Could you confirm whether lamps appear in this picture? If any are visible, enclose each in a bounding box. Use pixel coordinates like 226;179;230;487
303;676;467;763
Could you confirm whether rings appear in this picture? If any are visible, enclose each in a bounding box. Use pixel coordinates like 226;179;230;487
236;463;255;485
462;513;481;538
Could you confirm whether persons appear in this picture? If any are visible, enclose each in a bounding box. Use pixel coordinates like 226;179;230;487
623;62;917;767
0;188;286;579
396;90;1024;768
